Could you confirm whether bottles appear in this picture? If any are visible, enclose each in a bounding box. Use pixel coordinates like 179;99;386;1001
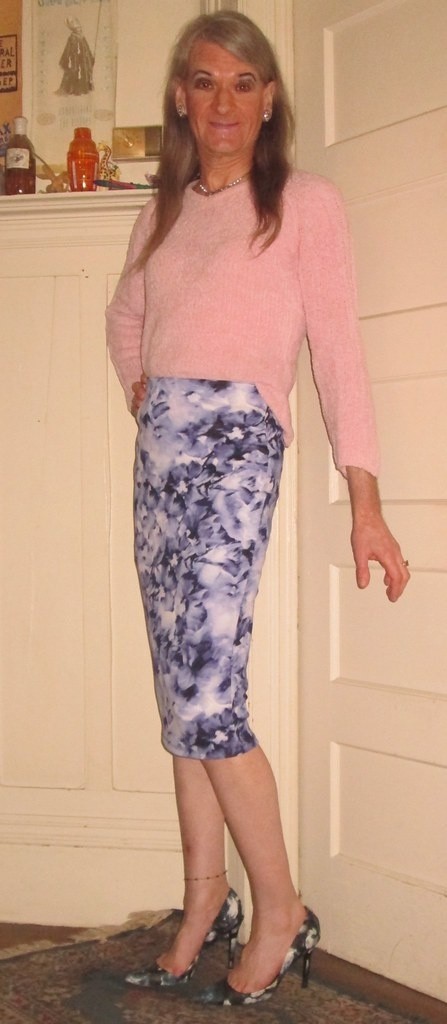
66;127;100;193
4;117;36;197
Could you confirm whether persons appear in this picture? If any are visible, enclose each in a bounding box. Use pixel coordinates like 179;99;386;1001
104;10;412;1005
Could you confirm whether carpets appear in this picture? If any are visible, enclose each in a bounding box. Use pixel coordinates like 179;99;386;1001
0;907;430;1024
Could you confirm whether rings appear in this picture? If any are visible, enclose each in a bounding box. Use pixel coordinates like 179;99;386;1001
403;560;408;566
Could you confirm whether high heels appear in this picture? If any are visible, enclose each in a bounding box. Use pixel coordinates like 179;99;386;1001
189;905;320;1006
125;888;244;991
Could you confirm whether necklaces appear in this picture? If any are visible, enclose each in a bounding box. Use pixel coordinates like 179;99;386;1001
199;176;243;196
